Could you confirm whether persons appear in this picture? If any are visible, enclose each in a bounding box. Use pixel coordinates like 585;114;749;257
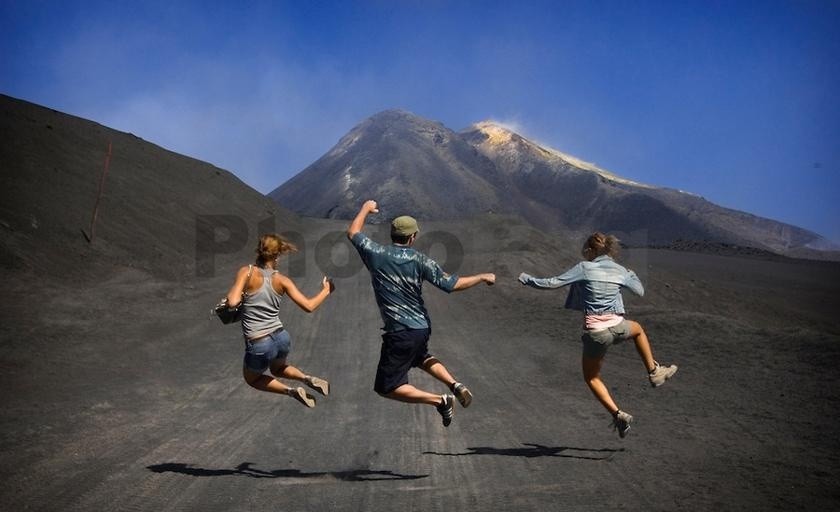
223;231;337;409
515;230;679;440
345;198;496;429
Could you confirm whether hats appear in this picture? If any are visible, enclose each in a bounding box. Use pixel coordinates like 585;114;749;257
390;215;420;236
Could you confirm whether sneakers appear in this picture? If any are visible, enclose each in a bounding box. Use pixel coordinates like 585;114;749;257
292;386;317;408
614;410;634;439
304;376;329;396
453;383;472;407
437;394;455;427
649;363;678;387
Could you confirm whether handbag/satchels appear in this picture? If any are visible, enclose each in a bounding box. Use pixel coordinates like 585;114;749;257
216;295;243;324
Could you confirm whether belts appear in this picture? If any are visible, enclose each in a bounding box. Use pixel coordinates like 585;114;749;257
247;326;283;343
